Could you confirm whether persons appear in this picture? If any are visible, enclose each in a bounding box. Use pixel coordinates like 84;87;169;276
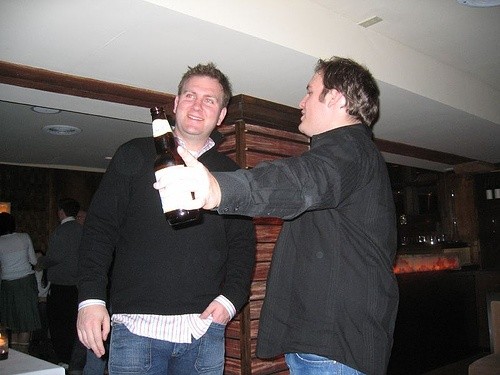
0;210;44;356
76;64;259;375
74;208;88;225
155;55;394;375
82;331;109;375
40;195;84;372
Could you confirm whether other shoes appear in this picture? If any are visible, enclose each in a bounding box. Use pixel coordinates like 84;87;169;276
58;362;69;370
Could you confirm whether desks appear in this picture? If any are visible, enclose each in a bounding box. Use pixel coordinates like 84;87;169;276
37;296;63;355
0;348;66;375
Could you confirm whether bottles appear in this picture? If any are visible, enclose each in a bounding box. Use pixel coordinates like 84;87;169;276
150;104;200;225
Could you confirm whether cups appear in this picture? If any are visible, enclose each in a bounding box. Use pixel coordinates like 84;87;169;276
0;323;9;360
